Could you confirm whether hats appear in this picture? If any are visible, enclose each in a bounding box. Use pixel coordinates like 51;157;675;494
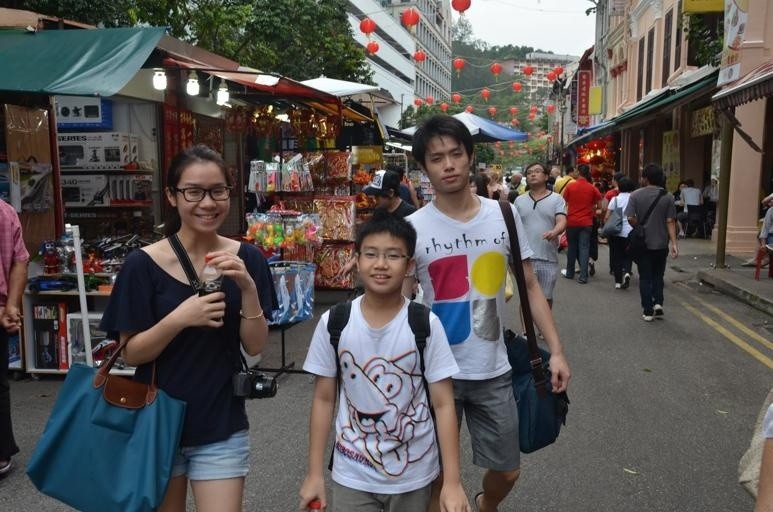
362;168;400;194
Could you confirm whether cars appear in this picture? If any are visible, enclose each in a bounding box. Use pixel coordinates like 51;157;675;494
511;166;523;175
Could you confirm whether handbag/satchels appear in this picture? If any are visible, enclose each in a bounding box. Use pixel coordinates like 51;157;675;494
502;326;570;454
24;361;187;512
624;222;647;265
599;207;623;239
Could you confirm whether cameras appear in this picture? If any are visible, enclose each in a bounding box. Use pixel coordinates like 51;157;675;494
233;370;277;399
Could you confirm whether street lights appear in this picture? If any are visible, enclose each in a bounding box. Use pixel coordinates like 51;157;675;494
557;96;568;177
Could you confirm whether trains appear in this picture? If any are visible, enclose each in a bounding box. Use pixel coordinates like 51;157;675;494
24;172;47;199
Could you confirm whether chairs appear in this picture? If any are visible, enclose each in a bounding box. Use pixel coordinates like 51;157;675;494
683;200;708;243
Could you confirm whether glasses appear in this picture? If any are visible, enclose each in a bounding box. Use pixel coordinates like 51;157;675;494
172;184;234;203
358;248;411;263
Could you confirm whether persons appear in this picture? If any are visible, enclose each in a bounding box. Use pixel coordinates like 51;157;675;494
95;138;280;512
0;197;31;480
296;209;473;512
672;179;703;238
704;178;719;202
744;378;772;512
470;167;524;202
362;162;420;219
740;194;772;267
551;164;603;283
624;163;678;321
397;113;571;512
514;163;567;341
603;175;634;289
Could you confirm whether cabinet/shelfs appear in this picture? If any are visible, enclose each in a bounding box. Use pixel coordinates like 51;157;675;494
52;167;155;236
21;288;138;385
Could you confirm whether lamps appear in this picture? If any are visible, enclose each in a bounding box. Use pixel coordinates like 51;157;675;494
151;63;167;92
184;66;200;98
214;77;232;110
343;95;377;122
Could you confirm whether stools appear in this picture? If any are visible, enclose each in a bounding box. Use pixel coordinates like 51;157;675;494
754;244;772;281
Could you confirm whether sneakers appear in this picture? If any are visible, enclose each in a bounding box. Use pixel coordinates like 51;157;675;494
620;273;630;289
643;304;664;321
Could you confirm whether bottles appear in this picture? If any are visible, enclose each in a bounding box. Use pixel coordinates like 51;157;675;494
199;255;221;324
307;499;322;511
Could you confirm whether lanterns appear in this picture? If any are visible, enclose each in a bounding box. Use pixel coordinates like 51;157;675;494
574;135;616;180
359;16;376;35
224;104;343;141
411;49;563;159
450;0;472;15
364;42;380;56
398;6;420;30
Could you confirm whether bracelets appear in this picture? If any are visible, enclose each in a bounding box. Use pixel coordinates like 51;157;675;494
237;307;265;322
